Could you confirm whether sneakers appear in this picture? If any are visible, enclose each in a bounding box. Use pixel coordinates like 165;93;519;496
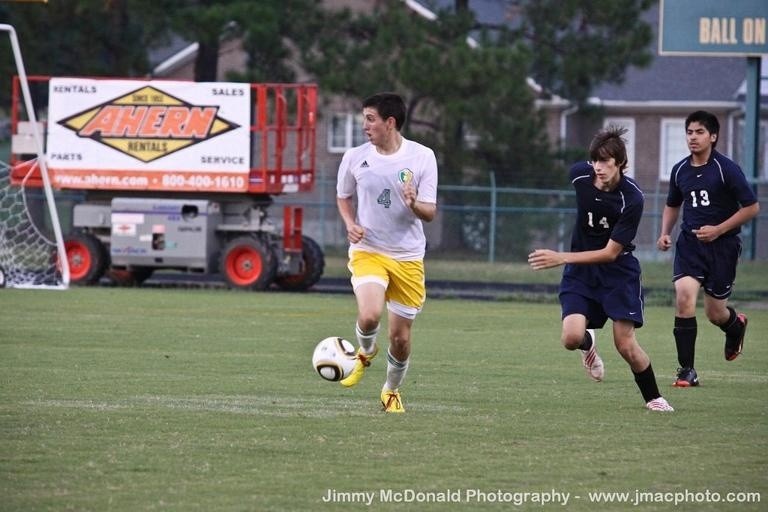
723;313;748;362
380;387;406;413
644;396;676;414
670;369;699;387
339;345;380;387
579;329;605;382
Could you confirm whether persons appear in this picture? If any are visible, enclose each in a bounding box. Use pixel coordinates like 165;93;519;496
528;123;674;412
656;110;760;387
336;94;437;414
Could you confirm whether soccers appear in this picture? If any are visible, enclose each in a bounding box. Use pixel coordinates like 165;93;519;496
312;336;357;382
